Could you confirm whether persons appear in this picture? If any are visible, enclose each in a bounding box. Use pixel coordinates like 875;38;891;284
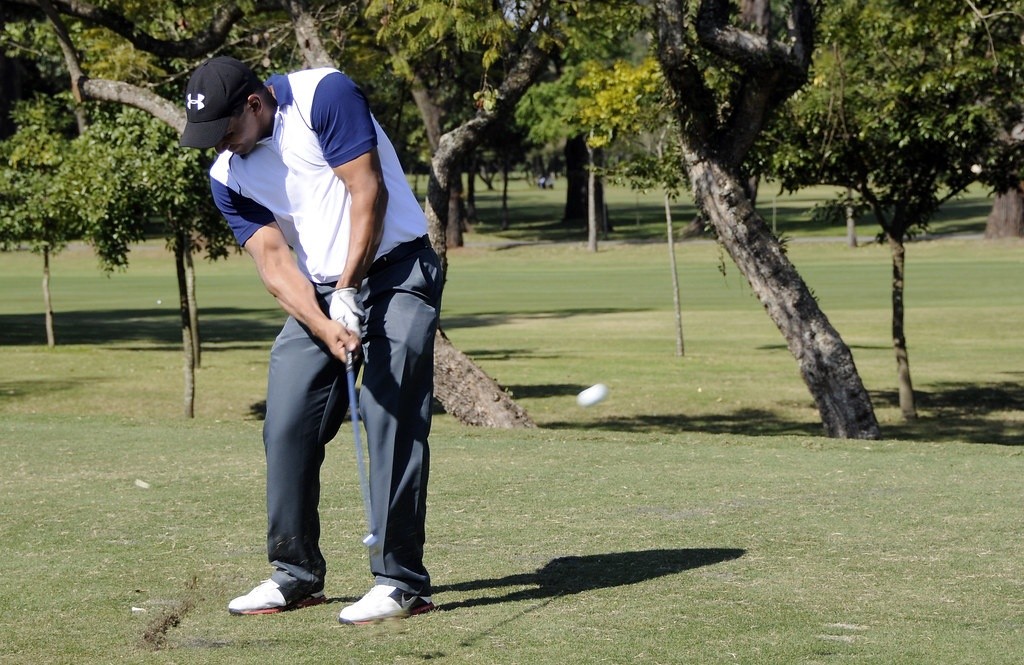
179;57;442;625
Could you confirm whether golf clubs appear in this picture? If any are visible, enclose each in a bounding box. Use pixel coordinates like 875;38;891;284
344;345;377;547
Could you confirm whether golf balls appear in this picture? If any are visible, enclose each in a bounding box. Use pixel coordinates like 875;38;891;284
576;383;610;407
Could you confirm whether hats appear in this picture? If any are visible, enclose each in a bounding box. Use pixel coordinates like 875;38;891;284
178;56;260;149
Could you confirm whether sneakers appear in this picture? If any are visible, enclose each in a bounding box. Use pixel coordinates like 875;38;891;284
227;579;329;614
338;583;434;627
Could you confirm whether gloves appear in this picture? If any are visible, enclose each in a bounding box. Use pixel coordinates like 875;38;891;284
329;286;367;350
